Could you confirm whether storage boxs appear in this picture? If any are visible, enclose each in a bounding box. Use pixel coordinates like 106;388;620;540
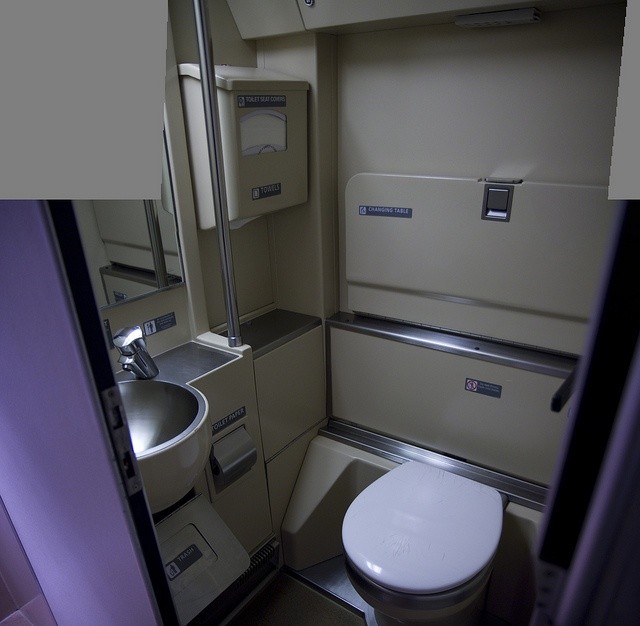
178;63;309;231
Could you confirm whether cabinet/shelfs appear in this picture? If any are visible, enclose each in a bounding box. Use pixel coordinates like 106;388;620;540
189;357;273;560
99;265;181;305
253;325;329;536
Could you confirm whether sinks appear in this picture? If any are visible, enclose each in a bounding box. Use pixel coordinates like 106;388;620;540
117;379;212;516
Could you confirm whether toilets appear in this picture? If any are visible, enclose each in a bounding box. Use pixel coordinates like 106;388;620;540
341;460;505;626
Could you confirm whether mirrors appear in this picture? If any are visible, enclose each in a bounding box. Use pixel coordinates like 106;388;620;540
71;131;186;312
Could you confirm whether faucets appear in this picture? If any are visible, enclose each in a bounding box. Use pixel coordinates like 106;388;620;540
112;325;159;380
